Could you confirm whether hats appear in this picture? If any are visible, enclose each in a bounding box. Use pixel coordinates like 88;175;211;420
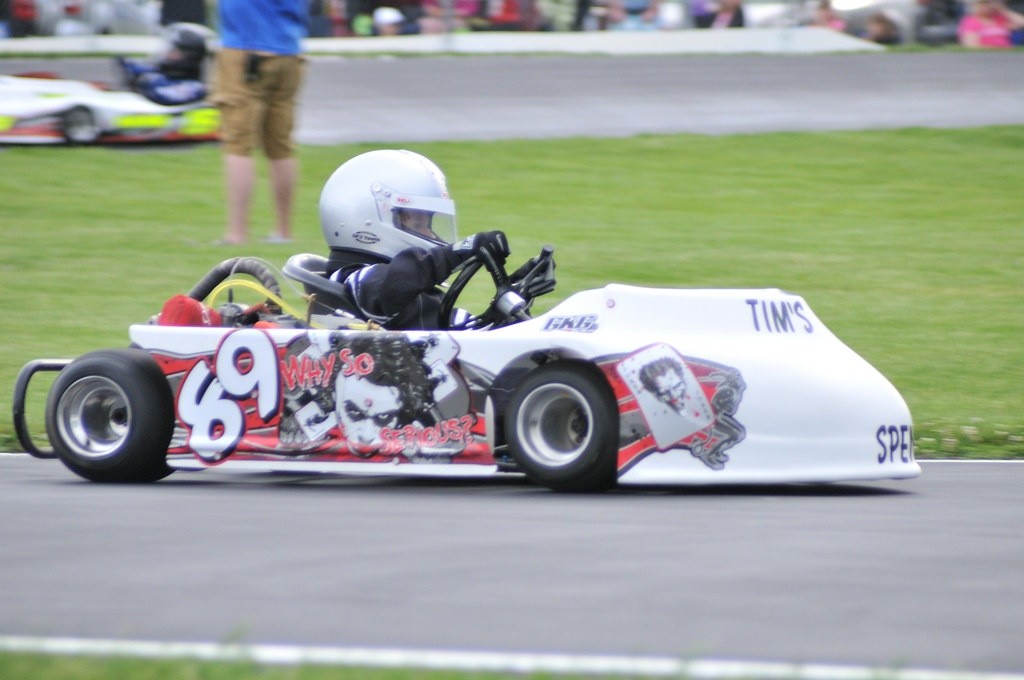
373;7;404;25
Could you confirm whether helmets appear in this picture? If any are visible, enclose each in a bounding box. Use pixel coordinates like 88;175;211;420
164;25;206;74
319;149;457;260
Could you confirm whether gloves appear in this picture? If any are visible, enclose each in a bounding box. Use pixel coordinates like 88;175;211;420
445;229;510;272
506;255;556;282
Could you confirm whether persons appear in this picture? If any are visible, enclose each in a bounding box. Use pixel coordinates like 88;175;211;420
212;0;308;247
284;148;557;328
0;0;1024;49
